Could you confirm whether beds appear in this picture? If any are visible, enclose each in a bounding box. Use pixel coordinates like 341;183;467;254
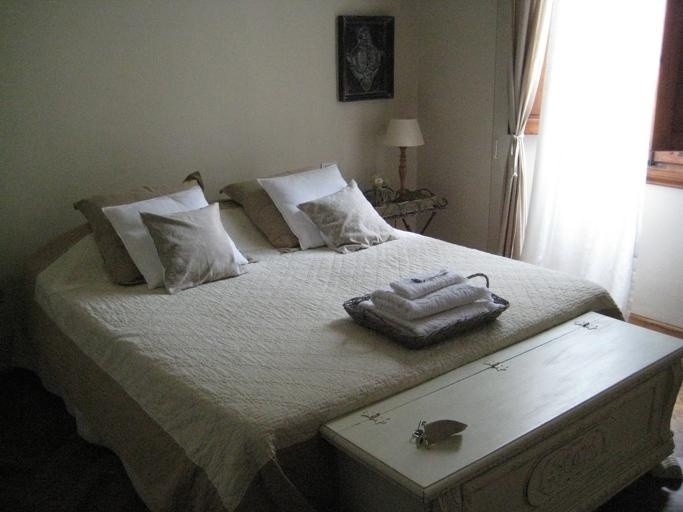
6;167;637;510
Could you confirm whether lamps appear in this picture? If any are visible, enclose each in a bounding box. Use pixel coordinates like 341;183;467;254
382;116;429;201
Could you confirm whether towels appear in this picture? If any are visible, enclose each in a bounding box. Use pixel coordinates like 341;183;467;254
370;283;482;319
389;271;465;296
354;289;492;336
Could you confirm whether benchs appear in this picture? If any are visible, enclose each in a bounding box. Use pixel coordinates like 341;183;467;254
317;304;681;508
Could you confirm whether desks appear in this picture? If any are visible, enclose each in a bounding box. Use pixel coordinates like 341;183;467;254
366;185;450;238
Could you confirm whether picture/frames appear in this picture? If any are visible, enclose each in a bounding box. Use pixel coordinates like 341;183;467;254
335;11;397;102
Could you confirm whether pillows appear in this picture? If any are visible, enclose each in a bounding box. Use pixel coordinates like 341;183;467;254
295;179;399;255
256;163;350;249
73;166;263;300
217;170;302;256
